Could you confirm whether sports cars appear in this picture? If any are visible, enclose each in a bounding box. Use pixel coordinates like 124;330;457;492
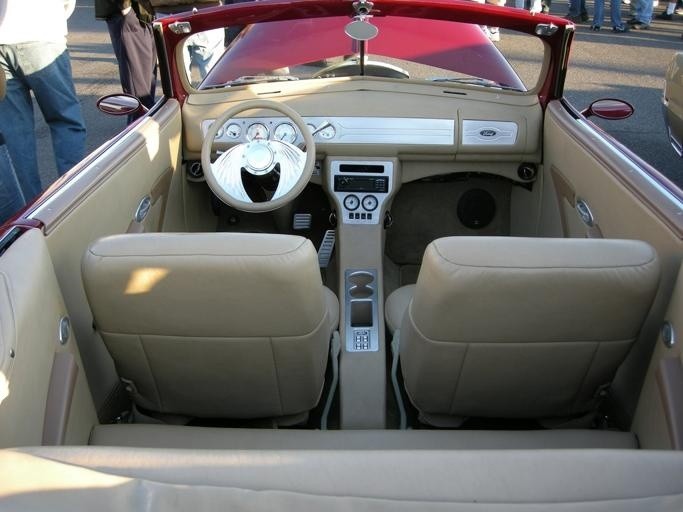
0;0;683;512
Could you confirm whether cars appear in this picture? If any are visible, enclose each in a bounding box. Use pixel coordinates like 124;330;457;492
660;50;683;166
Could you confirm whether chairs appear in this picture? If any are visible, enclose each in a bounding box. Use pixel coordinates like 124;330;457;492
384;235;661;430
80;232;339;428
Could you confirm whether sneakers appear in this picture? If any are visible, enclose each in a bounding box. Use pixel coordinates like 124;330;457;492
656;11;675;21
581;12;589;21
611;24;629;34
588;25;601;33
627;18;650;31
490;30;500;42
560;13;584;25
481;27;492;39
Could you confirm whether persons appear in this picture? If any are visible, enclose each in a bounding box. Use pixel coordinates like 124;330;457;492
152;0;225;98
474;0;682;43
0;1;87;207
0;64;28;228
94;0;157;126
